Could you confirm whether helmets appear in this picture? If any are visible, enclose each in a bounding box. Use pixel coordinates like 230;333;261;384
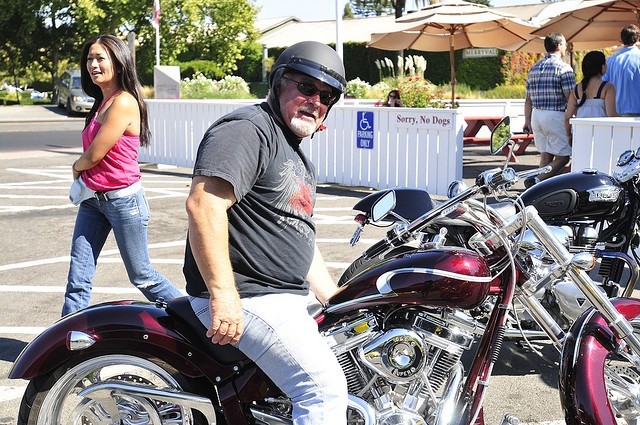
269;41;348;94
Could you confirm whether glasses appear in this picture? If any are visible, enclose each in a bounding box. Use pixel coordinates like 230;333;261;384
284;77;331;106
389;95;396;99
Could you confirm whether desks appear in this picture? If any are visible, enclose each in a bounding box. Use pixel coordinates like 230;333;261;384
463;117;507;137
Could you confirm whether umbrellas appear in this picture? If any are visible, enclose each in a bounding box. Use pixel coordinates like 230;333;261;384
531;0;639;51
366;1;541;110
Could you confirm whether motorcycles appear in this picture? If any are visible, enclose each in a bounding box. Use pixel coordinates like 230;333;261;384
8;115;640;425
337;147;640;347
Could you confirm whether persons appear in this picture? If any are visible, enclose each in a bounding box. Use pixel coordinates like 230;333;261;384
522;31;577;189
381;90;406;108
61;34;186;319
182;41;348;425
563;50;619;148
602;24;640;116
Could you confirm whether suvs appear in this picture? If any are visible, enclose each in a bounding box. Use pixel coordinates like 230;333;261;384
57;70;96;115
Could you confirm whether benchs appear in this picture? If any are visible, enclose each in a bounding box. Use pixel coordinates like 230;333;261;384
463;133;534;162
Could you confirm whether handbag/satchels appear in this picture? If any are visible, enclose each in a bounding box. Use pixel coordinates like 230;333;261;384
68;176;142;206
